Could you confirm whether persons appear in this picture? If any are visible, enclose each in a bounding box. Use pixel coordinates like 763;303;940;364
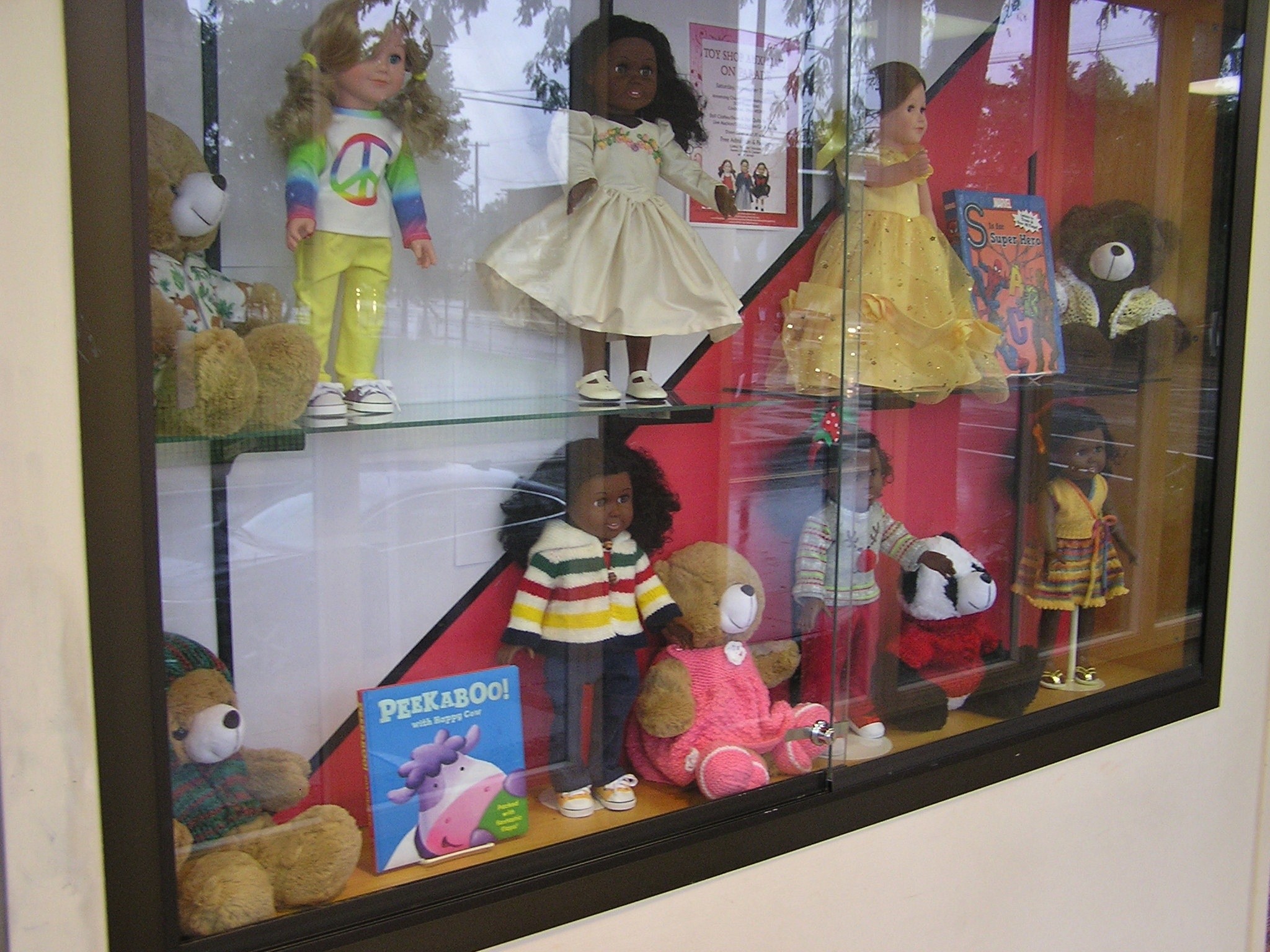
264;0;454;419
782;401;956;757
474;15;744;403
1010;401;1140;690
763;60;1010;405
497;438;695;817
717;159;770;212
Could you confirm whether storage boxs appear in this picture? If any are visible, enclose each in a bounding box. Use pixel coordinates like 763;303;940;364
941;188;1068;378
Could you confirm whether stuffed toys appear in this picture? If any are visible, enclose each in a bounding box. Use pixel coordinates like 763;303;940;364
868;529;1047;735
161;629;362;940
1054;199;1194;386
144;108;321;438
623;540;834;798
